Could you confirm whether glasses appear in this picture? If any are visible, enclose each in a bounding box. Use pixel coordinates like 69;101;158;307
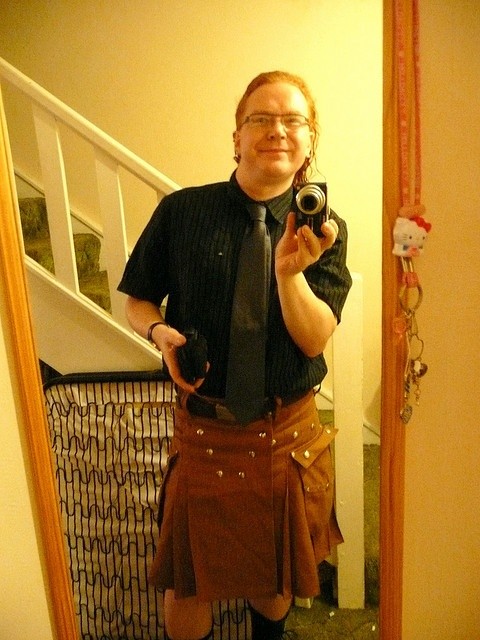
237;113;310;131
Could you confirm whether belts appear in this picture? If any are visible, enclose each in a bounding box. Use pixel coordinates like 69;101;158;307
187;390;310;424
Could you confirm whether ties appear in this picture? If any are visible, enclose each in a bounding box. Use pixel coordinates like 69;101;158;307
224;204;271;422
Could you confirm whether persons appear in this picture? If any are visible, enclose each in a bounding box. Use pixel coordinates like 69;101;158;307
123;72;353;640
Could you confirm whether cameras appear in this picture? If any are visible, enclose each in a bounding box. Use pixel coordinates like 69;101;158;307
292;179;330;243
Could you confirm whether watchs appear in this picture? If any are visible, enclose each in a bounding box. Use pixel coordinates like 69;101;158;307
145;321;162;346
